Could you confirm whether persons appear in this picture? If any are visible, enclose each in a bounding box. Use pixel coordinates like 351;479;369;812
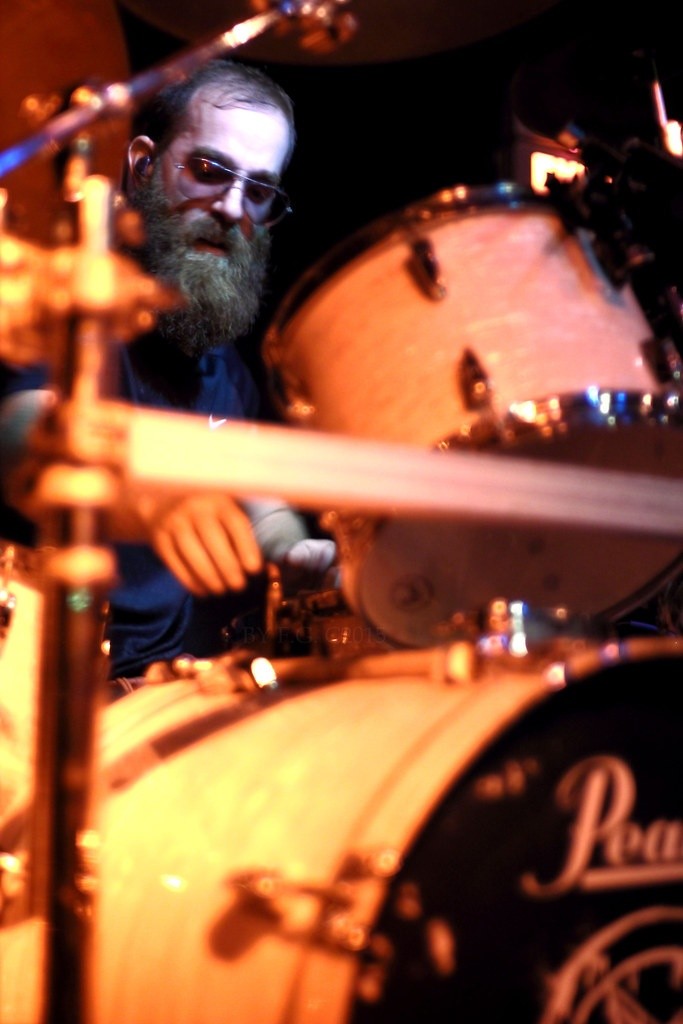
1;57;339;682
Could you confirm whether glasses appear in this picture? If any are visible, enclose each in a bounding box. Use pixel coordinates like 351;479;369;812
161;149;291;227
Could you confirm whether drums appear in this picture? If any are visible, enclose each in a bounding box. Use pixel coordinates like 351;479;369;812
0;181;683;1024
